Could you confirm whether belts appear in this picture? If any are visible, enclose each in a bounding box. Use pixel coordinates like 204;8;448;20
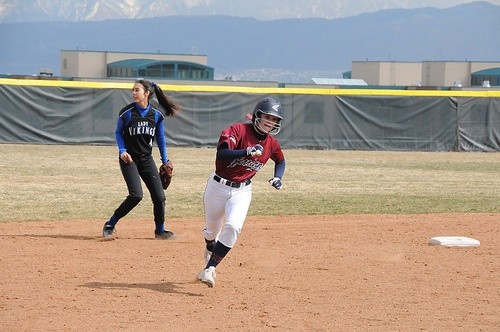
214;175;251;188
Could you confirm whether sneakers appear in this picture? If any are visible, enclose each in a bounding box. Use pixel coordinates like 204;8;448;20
154;228;175;240
103;221;115;240
204;244;213;265
197;266;216;288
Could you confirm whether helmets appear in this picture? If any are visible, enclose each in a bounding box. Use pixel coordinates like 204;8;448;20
251;96;284;124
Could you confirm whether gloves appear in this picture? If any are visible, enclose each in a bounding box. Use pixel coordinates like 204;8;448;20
268;176;283;190
246;143;264;157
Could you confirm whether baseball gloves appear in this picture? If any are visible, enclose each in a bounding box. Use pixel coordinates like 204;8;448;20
158;158;173;190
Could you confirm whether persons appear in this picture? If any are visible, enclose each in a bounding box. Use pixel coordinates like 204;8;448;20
102;81;180;240
197;98;285;288
